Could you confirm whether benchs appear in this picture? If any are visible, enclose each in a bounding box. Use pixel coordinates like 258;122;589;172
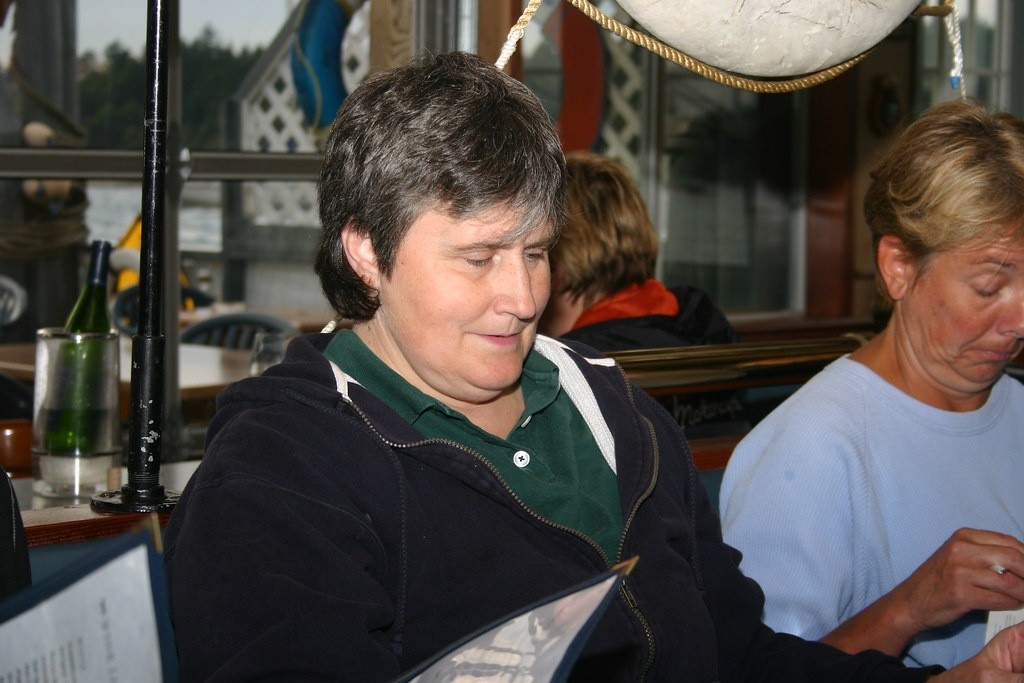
602;332;878;512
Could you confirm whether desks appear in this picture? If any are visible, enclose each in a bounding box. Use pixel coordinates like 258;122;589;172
0;337;251;425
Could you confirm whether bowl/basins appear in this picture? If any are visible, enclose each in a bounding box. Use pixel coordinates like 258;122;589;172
0;419;39;479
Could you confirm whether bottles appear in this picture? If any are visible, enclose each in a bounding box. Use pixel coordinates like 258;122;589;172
42;241;110;457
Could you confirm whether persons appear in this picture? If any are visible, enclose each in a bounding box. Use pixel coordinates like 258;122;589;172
163;51;1024;683
535;152;744;354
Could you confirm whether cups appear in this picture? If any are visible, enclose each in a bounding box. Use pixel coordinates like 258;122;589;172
250;334;300;376
30;328;121;497
